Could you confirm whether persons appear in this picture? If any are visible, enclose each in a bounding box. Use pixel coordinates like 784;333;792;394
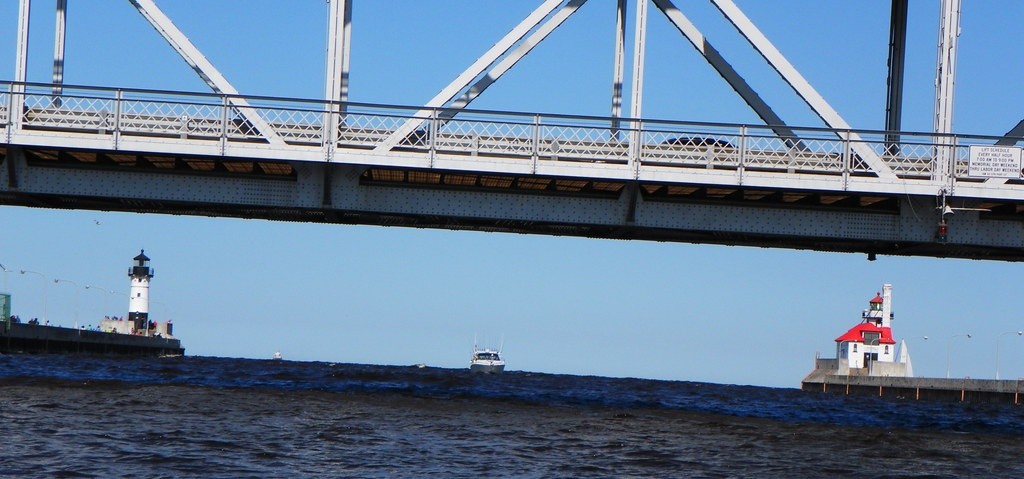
149;319;158;330
131;328;136;335
28;318;39;325
10;315;20;323
112;327;117;333
114;316;118;320
95;326;101;331
119;316;123;321
86;324;93;330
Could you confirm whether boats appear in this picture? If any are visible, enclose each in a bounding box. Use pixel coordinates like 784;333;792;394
272;351;282;360
470;334;506;373
